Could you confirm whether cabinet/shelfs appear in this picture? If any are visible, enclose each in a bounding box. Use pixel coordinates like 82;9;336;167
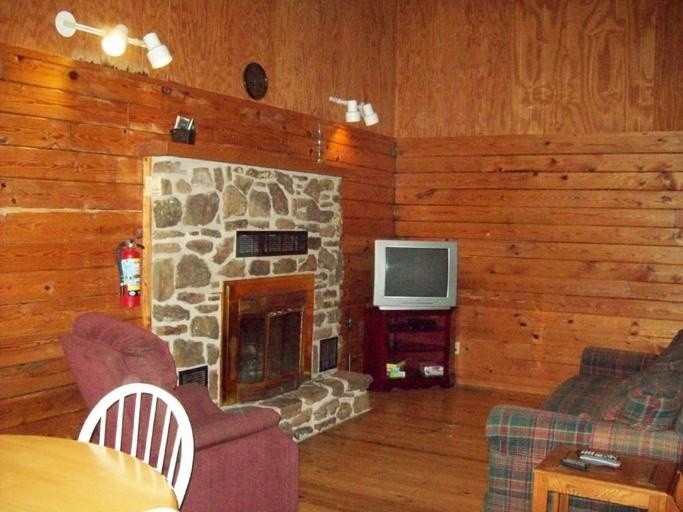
362;307;458;392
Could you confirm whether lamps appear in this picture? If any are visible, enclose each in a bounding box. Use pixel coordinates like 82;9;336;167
54;10;173;69
328;96;380;127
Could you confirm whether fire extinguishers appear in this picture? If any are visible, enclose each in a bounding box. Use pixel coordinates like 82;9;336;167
116;236;145;308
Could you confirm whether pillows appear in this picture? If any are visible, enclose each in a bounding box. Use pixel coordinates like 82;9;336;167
580;328;683;432
72;312;221;419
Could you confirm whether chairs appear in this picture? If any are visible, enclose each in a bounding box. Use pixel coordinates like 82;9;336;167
58;333;300;512
76;381;195;509
483;346;683;512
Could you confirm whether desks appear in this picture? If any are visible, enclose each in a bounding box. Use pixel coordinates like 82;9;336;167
0;433;179;512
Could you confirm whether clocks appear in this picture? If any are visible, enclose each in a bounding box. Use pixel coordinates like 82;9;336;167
242;62;269;100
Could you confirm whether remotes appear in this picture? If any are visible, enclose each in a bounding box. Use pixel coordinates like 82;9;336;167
579;455;622;468
576;448;620;460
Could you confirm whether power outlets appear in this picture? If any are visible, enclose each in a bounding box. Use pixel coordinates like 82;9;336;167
453;341;461;355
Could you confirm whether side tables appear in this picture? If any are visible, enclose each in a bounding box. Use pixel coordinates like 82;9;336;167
530;444;683;512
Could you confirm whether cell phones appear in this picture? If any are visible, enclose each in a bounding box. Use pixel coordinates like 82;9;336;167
560;457;587;469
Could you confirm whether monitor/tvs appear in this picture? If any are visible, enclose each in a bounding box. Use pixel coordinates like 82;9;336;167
373;239;458;310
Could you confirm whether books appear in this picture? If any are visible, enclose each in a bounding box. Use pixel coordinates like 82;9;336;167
384;359;445;378
